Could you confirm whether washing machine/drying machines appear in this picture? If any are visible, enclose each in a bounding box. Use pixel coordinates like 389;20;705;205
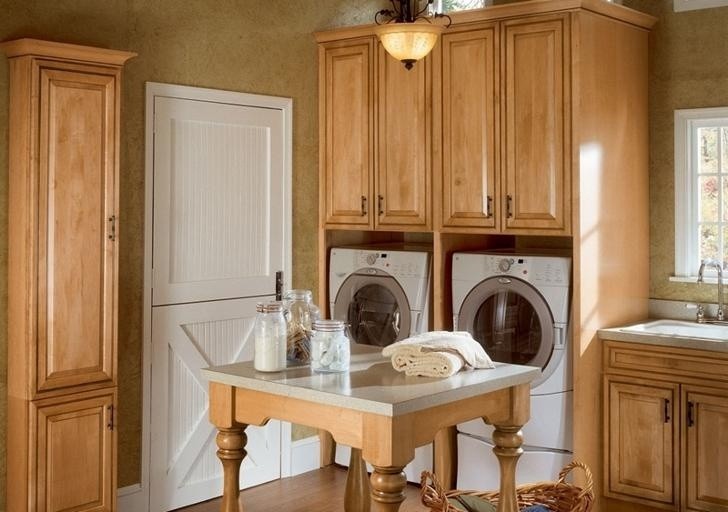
329;244;433;489
450;252;572;491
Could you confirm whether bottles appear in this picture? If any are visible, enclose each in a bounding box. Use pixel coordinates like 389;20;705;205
308;319;351;374
253;302;288;374
282;287;321;365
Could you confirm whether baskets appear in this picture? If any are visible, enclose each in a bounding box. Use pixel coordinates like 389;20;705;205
420;461;595;511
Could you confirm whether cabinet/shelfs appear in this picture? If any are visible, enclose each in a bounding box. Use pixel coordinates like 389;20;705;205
310;0;662;511
600;337;727;511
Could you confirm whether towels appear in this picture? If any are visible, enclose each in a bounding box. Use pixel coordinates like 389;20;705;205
380;330;495;379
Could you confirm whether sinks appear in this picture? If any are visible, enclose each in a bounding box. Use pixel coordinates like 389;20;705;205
621;318;728;341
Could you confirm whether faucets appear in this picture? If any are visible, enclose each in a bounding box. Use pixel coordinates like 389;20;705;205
696;259;724;321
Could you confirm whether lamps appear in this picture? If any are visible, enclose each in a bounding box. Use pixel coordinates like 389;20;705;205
369;1;452;70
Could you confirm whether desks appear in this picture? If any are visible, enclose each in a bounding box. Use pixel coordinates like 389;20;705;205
198;341;541;512
